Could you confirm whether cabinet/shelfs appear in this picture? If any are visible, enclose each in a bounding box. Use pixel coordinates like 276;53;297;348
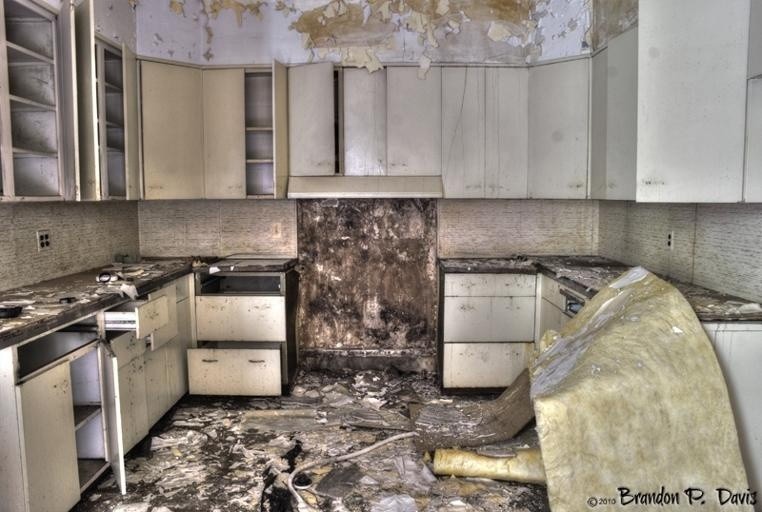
15;274;193;512
445;274;590;395
186;271;285;400
1;0;762;200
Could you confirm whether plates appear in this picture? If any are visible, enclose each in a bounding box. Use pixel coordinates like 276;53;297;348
186;271;285;400
445;274;590;395
